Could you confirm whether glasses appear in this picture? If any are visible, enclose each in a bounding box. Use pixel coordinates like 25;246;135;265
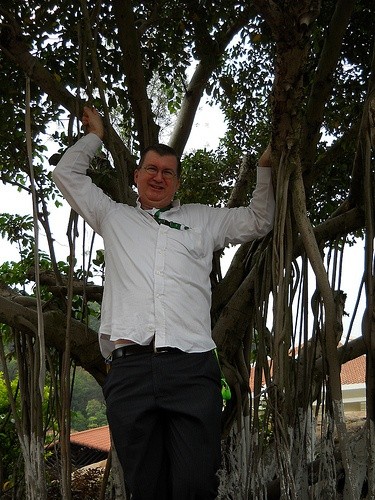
143;165;176;177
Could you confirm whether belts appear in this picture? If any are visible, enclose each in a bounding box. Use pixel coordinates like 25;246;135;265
110;345;169;359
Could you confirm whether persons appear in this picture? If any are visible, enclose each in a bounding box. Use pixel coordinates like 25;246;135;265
51;107;276;500
